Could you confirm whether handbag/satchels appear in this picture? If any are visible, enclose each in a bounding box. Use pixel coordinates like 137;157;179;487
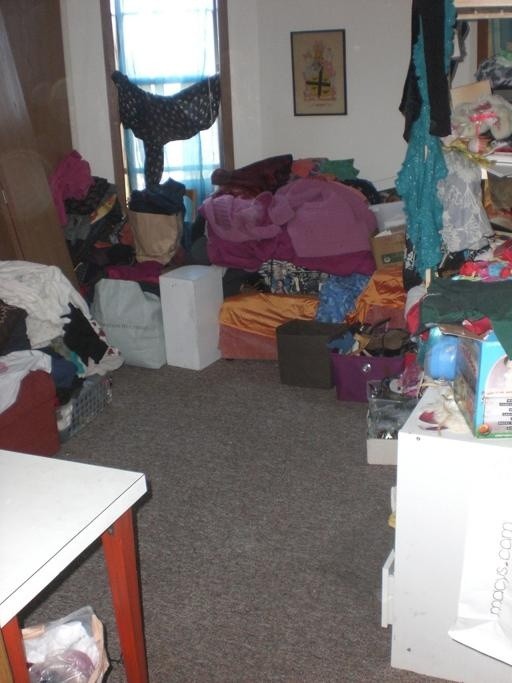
129;209;185;267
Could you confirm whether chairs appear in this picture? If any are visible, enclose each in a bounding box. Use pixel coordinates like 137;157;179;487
104;184;200;293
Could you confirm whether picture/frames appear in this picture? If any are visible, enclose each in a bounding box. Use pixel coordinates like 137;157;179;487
289;26;350;118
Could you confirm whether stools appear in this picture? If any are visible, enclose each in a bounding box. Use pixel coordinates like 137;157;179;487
0;366;63;458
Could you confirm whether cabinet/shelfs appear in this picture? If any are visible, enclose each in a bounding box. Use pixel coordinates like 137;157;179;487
1;0;83;313
376;377;512;683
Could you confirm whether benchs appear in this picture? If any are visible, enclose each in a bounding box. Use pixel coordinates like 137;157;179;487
215;286;406;364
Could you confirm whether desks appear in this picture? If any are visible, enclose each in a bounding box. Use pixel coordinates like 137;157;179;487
0;446;158;682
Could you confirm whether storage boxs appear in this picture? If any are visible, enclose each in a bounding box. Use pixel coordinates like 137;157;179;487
328;345;410;404
368;222;422;274
368;175;408;232
275;316;355;391
365;412;400;466
434;311;512;440
158;262;228;372
